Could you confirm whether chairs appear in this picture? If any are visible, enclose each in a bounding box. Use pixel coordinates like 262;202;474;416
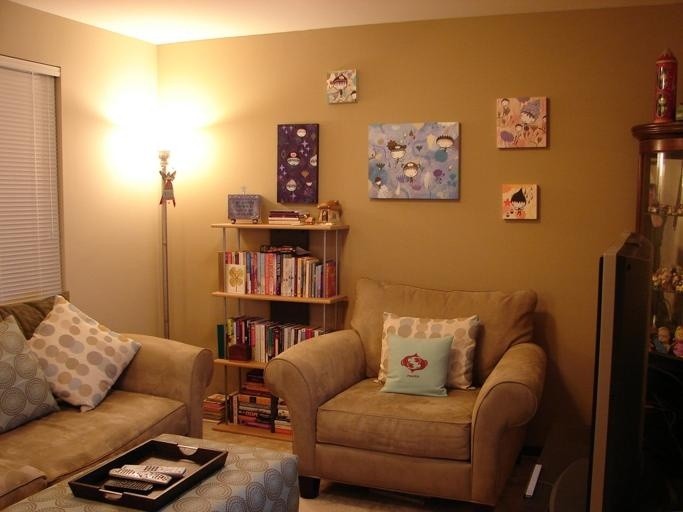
263;276;546;512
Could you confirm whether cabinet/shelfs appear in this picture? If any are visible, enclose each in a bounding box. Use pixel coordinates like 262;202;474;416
630;119;683;368
210;220;351;441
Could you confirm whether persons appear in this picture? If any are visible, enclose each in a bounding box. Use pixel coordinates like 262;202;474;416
653;326;671;351
671;326;683;358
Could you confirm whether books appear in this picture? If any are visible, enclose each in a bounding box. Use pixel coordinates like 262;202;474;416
201;209;336;434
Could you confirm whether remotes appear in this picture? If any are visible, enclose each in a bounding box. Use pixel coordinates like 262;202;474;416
121;464;186;478
108;467;172;487
104;480;154;495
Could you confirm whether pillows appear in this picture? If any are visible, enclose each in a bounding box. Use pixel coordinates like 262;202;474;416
376;311;480;390
26;294;143;411
380;331;454;397
0;291;70;340
0;315;60;435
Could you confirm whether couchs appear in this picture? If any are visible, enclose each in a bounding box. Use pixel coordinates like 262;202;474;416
0;293;214;512
